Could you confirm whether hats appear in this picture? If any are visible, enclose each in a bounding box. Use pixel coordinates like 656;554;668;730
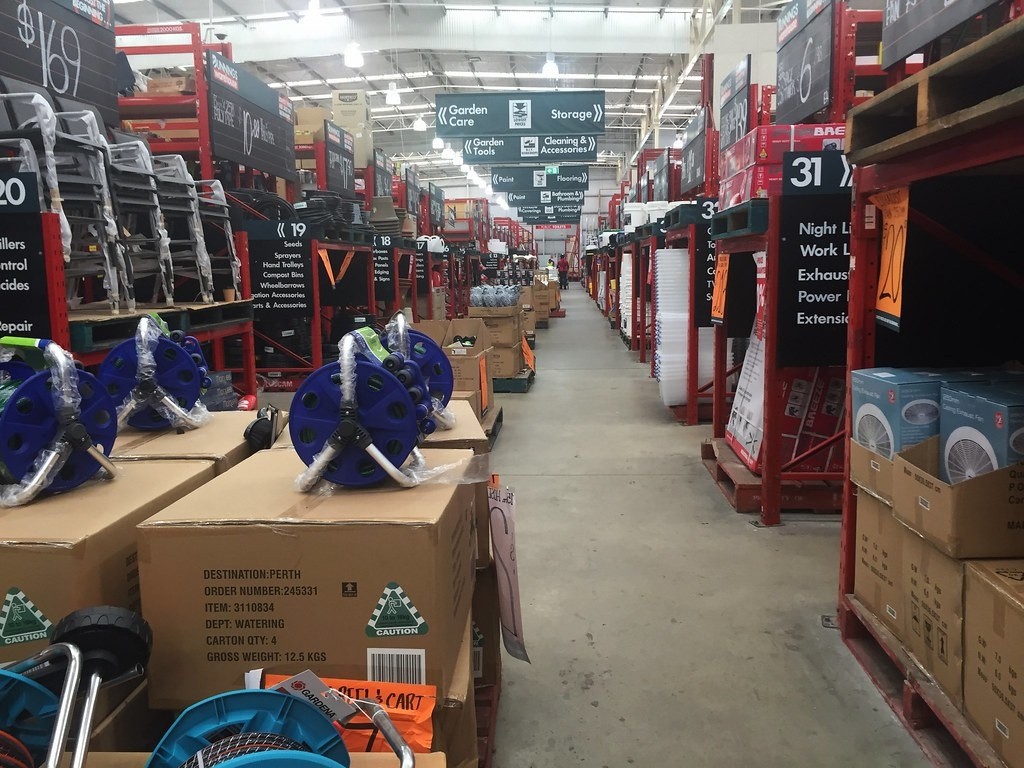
560;254;564;257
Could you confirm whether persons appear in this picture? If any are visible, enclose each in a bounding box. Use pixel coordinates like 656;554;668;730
547;259;554;266
557;253;569;289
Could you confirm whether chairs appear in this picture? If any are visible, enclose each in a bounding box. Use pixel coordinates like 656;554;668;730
0;74;243;313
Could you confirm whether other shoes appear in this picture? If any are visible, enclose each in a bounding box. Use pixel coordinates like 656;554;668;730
560;287;562;289
564;287;566;289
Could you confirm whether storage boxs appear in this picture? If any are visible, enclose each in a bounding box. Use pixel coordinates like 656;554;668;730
164;118;199;138
147;76;196;96
37;750;447;768
856;41;882;97
472;546;501;691
355;179;365;190
302;159;317;169
850;366;970;461
296;159;301;169
96;409;290;475
0;459;217;739
939;380;1024;485
134;449;478;711
432;608;479;768
850;437;894;508
404;281;560;424
905;528;966;714
853;486;906;644
119;118;163;139
892;434;1024;559
963;559;1024;768
652;249;736;407
295;133;314;145
267;398;491;569
